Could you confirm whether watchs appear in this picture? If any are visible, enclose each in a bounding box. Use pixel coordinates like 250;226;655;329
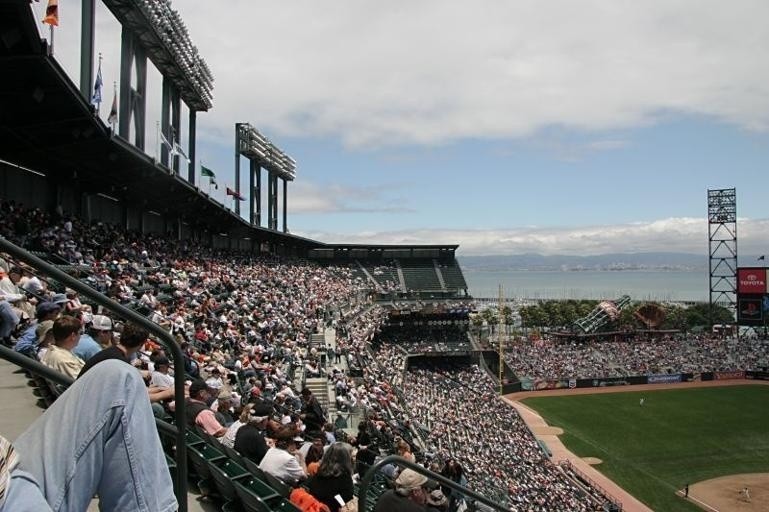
685;484;689;499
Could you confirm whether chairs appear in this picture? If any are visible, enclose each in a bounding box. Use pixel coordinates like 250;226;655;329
16;246;621;512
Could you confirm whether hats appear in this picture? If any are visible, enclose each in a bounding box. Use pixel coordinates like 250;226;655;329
189;369;274;416
88;314;112;331
396;465;447;505
153;357;174;366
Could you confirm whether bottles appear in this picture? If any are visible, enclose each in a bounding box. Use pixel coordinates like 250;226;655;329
570;295;631;333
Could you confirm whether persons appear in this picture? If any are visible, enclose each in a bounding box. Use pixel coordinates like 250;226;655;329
733;488;753;505
481;321;769;383
480;368;623;512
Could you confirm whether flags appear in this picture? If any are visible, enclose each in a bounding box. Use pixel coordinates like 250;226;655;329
172;141;192;165
232;195;248;201
91;65;104;104
226;187;240;197
209;177;218;189
107;94;118;124
157;129;173;155
41;0;60;28
201;166;216;178
